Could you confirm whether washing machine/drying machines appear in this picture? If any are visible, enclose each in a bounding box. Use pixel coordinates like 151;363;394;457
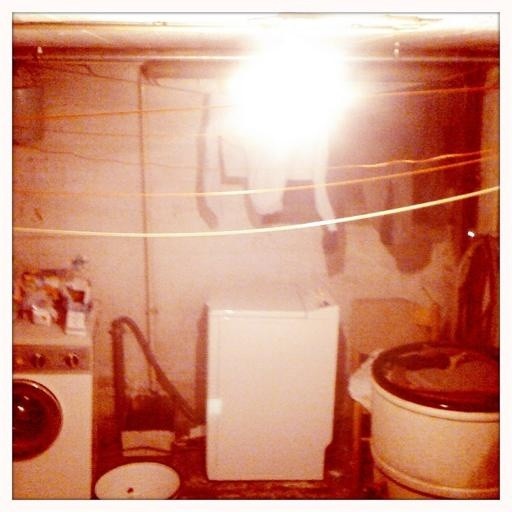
205;281;341;480
12;311;96;500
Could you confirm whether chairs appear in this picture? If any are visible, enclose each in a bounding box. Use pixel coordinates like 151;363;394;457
348;295;421;489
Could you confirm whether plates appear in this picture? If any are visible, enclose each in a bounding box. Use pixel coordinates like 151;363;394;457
93;459;183;503
